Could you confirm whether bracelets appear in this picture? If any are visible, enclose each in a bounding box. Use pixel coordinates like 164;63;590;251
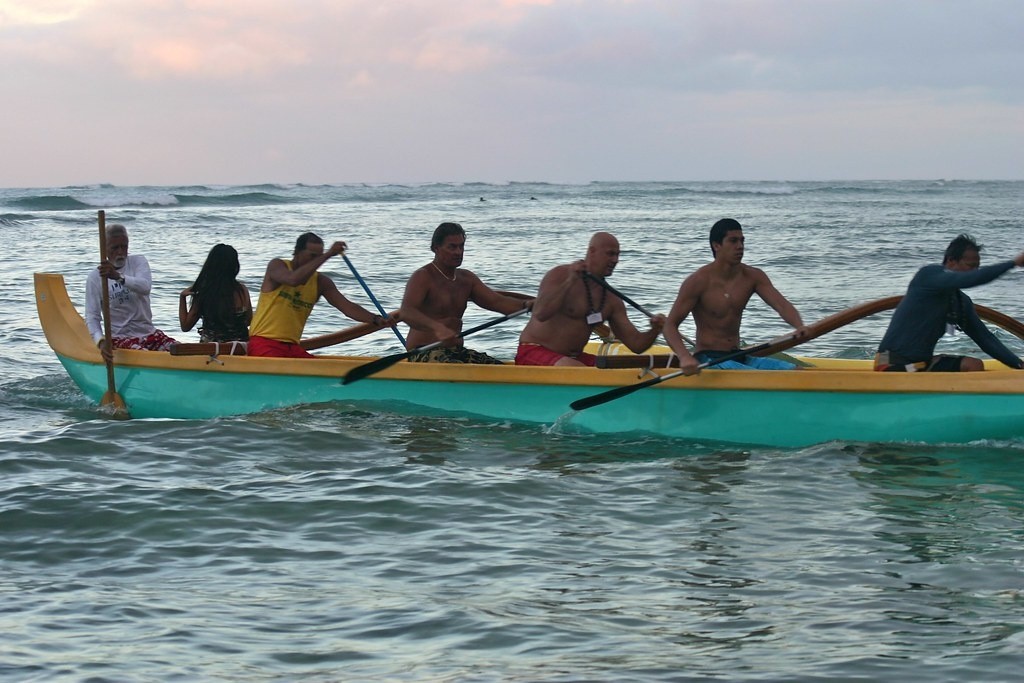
523;300;528;309
372;314;381;325
117;273;124;283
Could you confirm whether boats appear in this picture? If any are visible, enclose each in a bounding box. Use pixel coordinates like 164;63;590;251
29;268;1024;444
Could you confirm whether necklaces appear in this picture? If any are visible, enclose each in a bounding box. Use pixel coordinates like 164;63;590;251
431;261;456;281
722;286;730;298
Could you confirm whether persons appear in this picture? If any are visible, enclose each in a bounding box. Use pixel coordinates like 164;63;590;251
874;234;1024;373
662;218;809;376
515;232;666;367
399;223;535;366
84;224;180;361
248;232;396;359
179;243;253;343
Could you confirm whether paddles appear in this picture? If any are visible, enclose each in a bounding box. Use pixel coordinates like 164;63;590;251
340;303;533;386
569;331;808;412
335;243;408;351
572;260;696;349
97;209;129;420
